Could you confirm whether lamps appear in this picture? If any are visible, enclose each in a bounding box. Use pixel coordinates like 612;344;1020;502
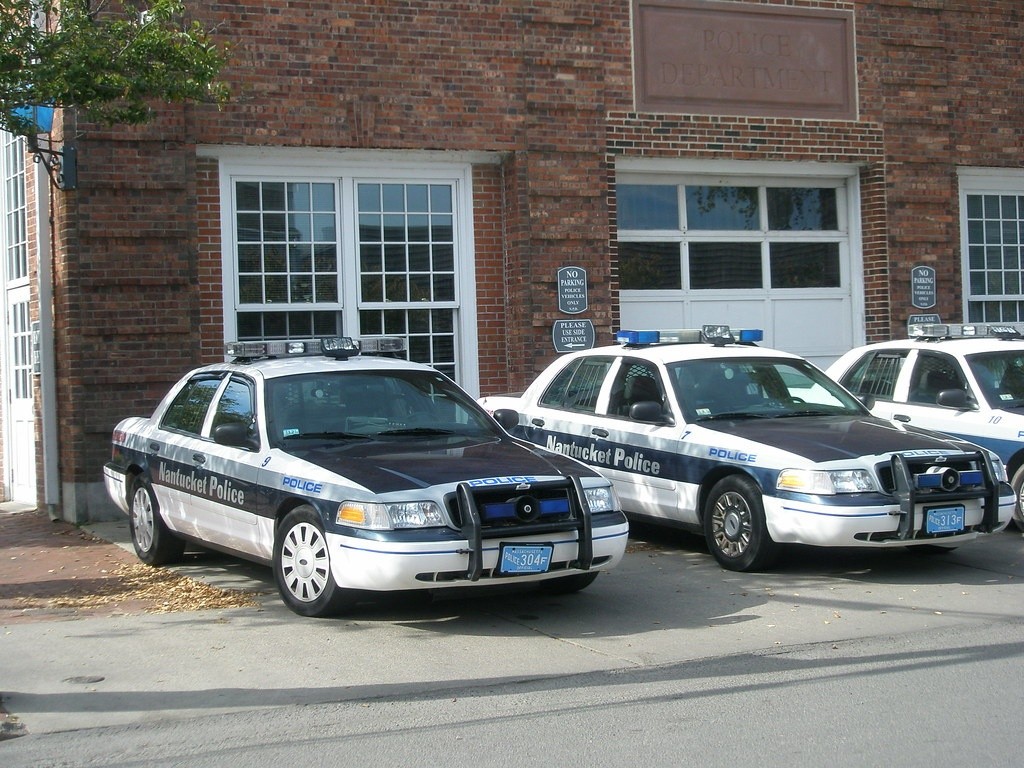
702;325;735;347
989;324;1021;341
28;133;66;189
320;336;360;361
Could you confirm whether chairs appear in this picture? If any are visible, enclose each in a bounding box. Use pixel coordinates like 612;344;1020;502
621;377;669;418
920;371;965;406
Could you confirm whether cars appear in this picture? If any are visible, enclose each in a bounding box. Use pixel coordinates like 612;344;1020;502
102;335;630;618
472;325;1018;573
809;324;1024;533
